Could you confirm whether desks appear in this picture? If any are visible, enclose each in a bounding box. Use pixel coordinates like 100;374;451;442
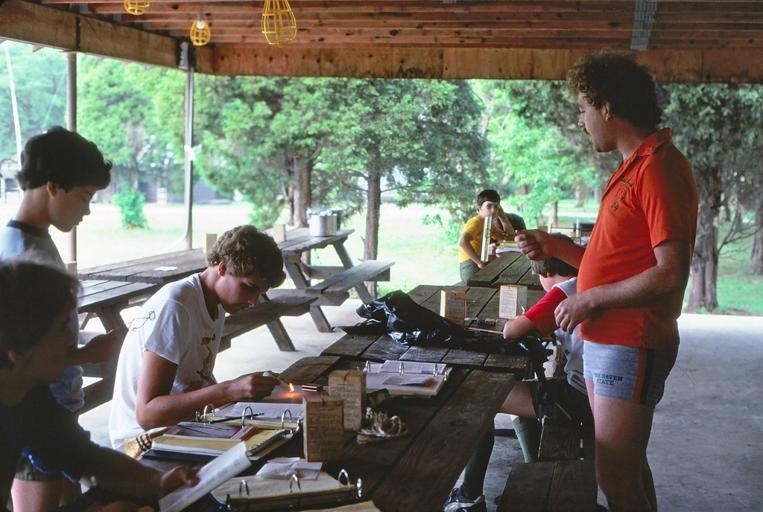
73;241;315;357
320;285;571;370
470;249;567;285
276;225;397;334
57;280;156;365
55;360;521;510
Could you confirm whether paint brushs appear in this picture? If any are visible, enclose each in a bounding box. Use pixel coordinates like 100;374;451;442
209;413;264;424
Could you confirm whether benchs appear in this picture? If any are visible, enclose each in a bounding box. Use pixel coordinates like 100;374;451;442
534;347;593;460
497;459;598;512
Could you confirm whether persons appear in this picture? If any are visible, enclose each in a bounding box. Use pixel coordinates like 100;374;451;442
453;189;517;286
1;123;125;512
0;256;204;512
441;233;586;512
510;44;700;512
105;223;287;450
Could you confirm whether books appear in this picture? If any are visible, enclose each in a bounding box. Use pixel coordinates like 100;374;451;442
140;358;455;511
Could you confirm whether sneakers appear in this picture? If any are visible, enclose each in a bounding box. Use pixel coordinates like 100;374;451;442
439;485;488;511
